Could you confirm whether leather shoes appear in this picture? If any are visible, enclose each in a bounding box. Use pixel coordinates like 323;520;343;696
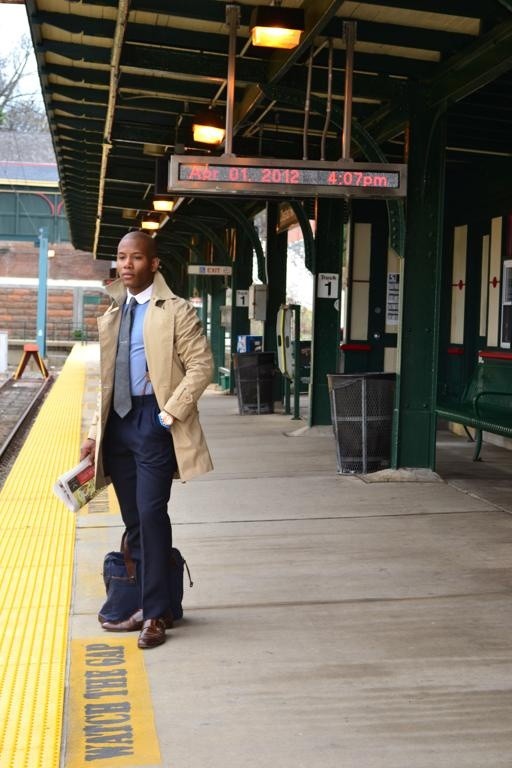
102;608;174;632
138;617;165;648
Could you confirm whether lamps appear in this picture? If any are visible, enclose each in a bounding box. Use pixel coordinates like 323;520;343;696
250;4;305;49
140;111;226;230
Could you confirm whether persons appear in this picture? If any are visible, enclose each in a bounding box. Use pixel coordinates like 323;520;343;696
79;230;215;649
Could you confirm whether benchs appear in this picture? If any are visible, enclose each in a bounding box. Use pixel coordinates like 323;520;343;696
435;363;512;461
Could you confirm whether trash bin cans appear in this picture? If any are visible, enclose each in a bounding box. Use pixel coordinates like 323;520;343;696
232;352;277;415
326;373;397;475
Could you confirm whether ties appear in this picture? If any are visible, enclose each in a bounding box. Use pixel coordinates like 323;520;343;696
112;298;135;419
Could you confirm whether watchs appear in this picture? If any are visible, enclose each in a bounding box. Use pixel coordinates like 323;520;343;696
159;411;176;427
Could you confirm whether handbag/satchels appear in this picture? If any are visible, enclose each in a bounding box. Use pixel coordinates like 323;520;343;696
98;528;186;623
